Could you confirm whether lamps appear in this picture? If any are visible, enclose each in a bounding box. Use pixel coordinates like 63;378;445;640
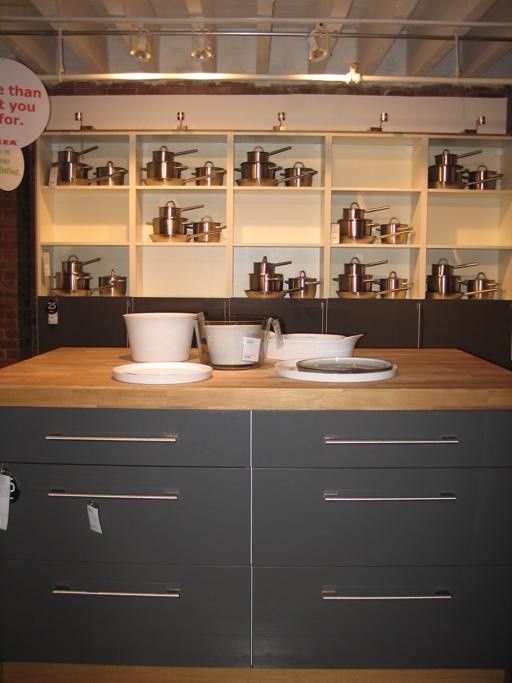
127;24;154;62
304;29;330;61
190;28;213;62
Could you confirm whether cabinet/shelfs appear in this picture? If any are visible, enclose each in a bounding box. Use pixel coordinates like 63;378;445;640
36;129;512;298
0;409;251;672
251;409;512;674
35;299;510;357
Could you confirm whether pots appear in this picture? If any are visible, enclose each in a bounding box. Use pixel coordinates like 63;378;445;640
333;257;414;300
244;255;322;299
51;254;128;296
337;201;413;245
430;148;505;190
144;200;228;244
426;257;502;299
234;146;318;188
141;145;227;186
47;145;129;188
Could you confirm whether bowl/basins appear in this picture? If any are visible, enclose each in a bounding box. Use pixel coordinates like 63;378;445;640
123;311;364;371
